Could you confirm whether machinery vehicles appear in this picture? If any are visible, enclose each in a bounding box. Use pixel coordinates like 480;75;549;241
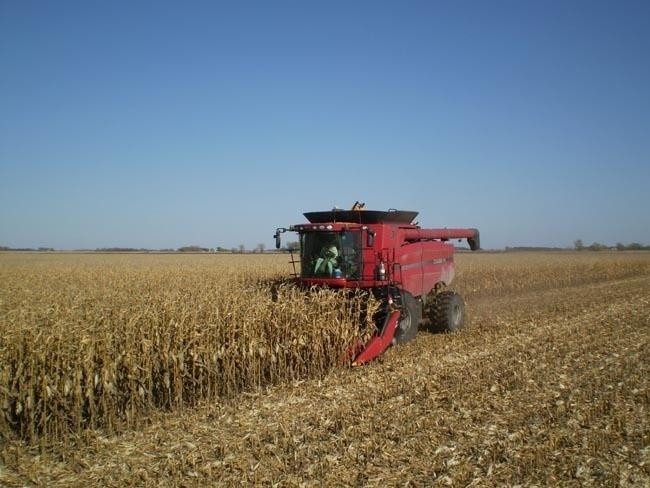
270;203;480;370
